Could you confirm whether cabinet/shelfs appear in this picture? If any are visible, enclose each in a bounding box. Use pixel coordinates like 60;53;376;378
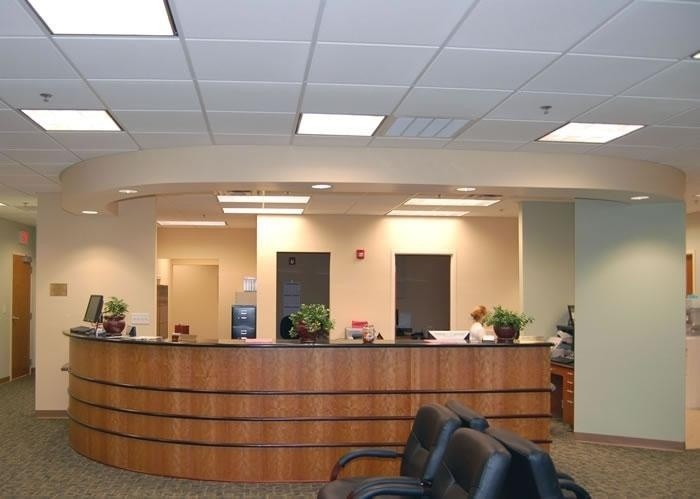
231;286;257;339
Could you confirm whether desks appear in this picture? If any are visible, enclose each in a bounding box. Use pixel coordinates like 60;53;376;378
551;364;574;432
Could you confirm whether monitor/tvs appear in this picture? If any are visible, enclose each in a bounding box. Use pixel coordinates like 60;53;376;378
126;326;136;336
82;295;103;323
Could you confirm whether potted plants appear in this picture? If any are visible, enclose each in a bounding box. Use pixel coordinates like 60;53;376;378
103;297;129;336
486;306;535;343
288;304;334;343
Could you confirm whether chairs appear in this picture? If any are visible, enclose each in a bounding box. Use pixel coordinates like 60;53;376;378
318;400;591;499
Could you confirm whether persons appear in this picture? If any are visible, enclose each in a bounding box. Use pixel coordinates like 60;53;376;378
469;305;488;341
